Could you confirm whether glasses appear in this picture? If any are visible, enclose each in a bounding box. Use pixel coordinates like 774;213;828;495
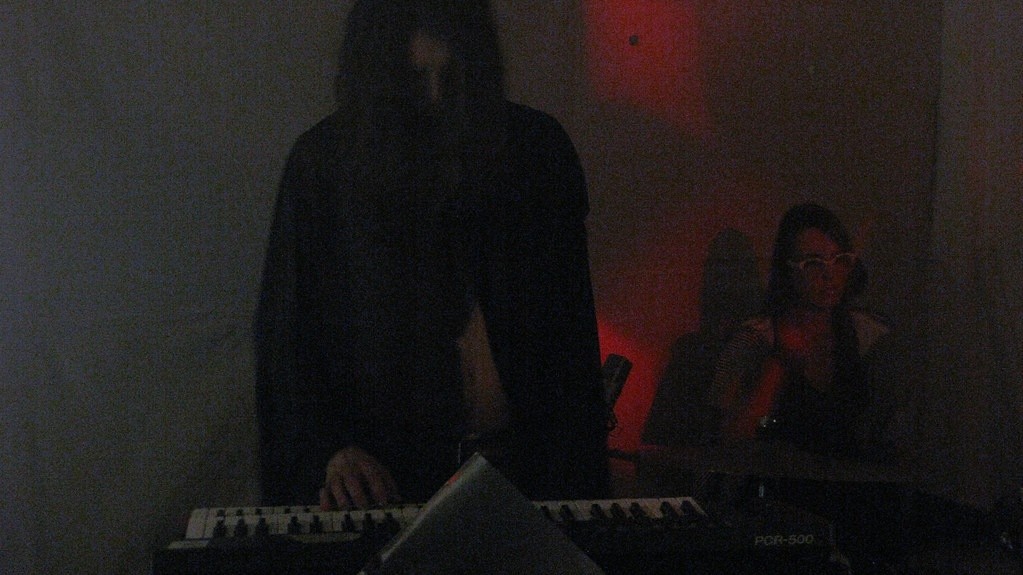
790;252;856;276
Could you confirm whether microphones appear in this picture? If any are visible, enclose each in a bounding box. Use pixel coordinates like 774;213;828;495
567;354;632;462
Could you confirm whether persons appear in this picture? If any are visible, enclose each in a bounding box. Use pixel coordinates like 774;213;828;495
251;0;605;511
710;201;897;460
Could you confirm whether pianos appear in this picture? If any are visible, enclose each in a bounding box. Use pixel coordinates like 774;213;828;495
156;457;740;573
